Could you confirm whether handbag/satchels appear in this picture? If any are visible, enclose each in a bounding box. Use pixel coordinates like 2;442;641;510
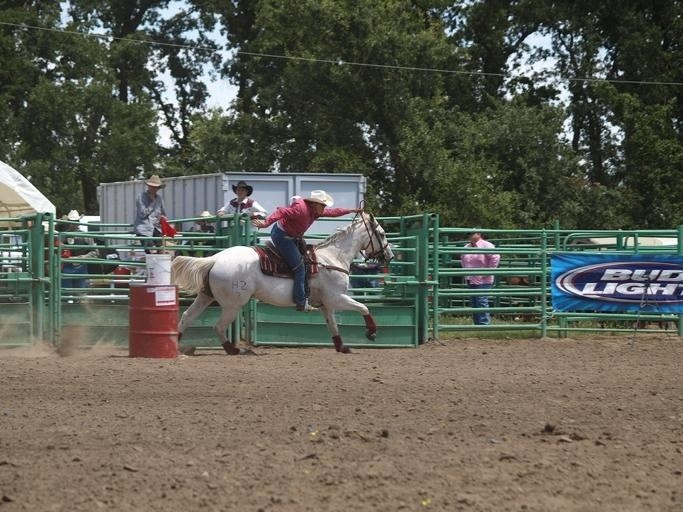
88;246;122;276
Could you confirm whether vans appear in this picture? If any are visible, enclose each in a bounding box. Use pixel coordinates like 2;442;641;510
41;214;101;234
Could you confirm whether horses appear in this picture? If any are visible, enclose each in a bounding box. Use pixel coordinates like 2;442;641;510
171;210;395;355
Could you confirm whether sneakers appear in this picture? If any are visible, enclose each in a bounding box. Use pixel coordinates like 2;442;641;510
296;303;320;311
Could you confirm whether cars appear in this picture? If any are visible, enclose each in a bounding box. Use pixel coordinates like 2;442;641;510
0;234;29;271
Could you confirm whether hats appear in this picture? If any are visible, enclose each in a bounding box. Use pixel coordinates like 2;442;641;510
144;175;166;189
232;181;253;196
62;210;84;221
303;190;335;208
195;211;217;224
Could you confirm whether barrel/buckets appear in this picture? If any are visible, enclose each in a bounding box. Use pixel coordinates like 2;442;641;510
119;251;147;273
114;269;130;288
128;284;179;358
146;255;172;285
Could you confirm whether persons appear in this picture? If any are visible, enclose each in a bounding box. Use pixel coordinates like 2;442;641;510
251;188;364;311
51;211;217;303
131;175;167;255
218;181;266;245
460;226;501;325
